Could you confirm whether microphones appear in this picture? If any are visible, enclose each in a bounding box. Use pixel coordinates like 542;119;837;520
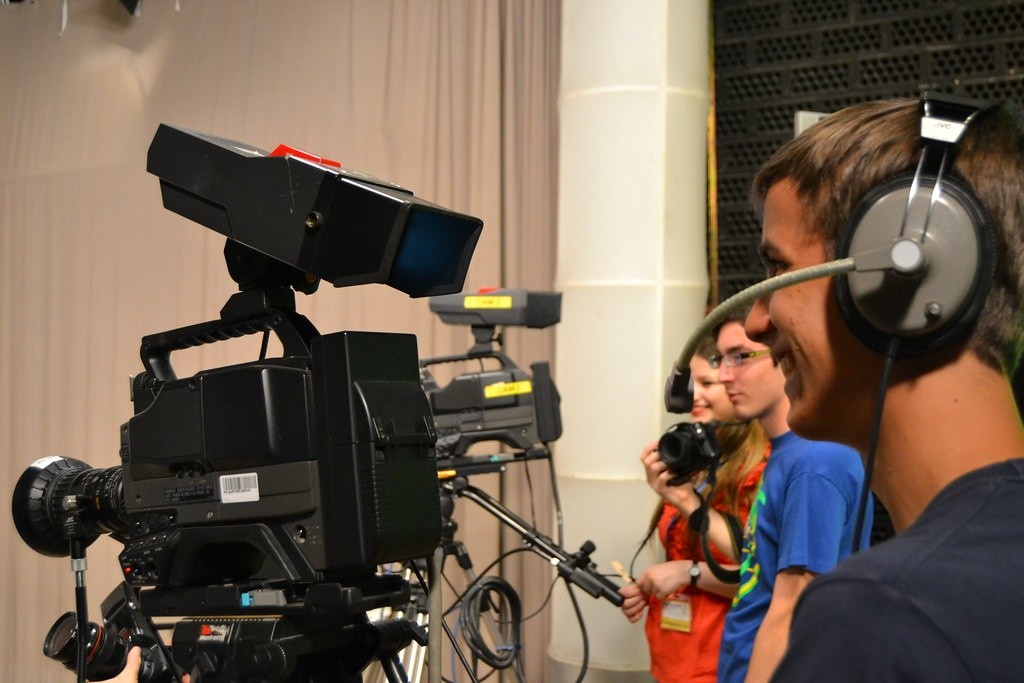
664;257;854;414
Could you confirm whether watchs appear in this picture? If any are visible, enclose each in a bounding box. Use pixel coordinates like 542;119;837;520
689;559;700;590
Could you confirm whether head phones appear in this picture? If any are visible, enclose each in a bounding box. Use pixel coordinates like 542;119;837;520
832;92;1000;358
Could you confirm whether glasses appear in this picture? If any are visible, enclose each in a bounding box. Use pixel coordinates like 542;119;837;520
707;348;772;368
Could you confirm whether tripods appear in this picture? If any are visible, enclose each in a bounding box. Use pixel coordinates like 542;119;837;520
362;473;624;683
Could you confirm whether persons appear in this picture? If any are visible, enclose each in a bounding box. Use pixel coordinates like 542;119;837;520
616;304;873;683
744;96;1024;683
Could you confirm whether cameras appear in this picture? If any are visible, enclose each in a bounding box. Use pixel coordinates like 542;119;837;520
653;421;722;487
